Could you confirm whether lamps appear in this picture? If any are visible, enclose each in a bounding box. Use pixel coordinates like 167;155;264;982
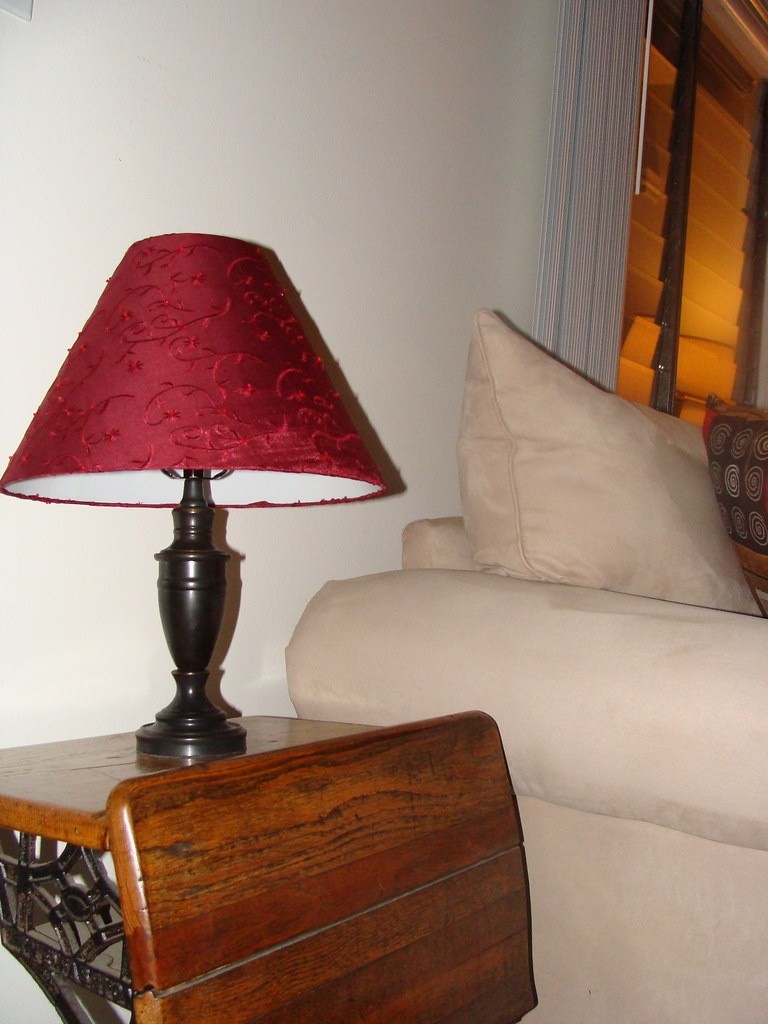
0;232;405;771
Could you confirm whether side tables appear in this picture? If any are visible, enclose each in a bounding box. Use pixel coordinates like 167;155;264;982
0;716;387;1024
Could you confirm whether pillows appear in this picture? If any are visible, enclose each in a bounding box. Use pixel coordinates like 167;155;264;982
702;393;768;619
454;305;764;619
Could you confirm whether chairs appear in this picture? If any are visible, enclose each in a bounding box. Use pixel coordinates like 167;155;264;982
104;705;540;1024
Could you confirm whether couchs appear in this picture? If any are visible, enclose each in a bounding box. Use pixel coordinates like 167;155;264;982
284;516;768;1024
